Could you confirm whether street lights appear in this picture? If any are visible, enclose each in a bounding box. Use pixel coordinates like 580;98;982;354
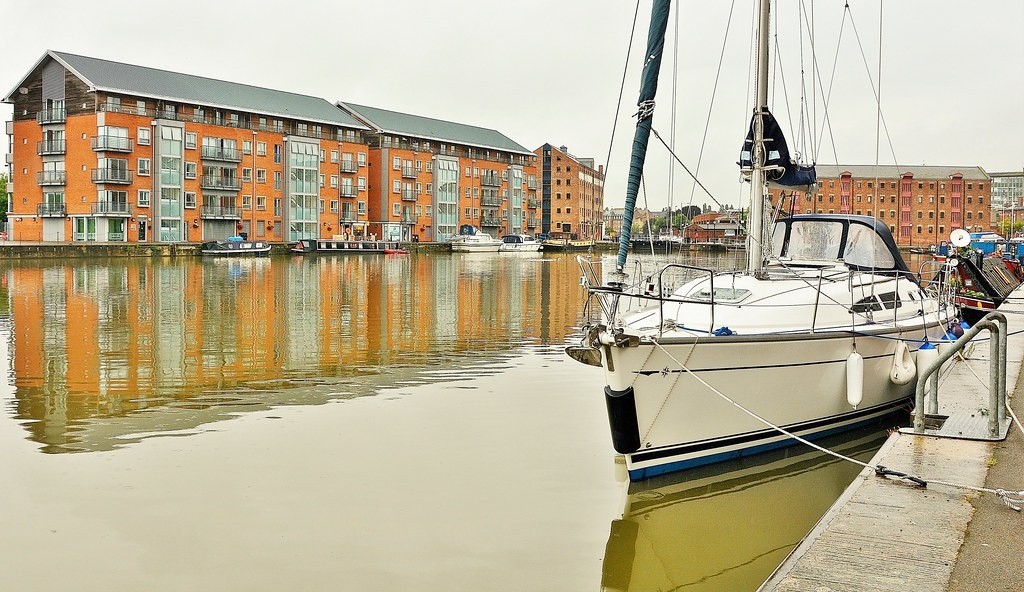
1011;202;1015;238
675;206;677;225
713;221;716;243
707;222;709;242
741;219;744;234
1002;202;1006;234
681;203;684;229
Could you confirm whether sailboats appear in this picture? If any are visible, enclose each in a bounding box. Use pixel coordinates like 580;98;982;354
564;0;962;483
601;417;912;591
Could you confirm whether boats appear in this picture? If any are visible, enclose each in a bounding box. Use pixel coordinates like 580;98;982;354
909;244;937;254
539;232;596;252
498;234;542;252
291;238;400;255
201;237;272;254
450;233;504;252
384;249;411;254
925;251;1023;312
